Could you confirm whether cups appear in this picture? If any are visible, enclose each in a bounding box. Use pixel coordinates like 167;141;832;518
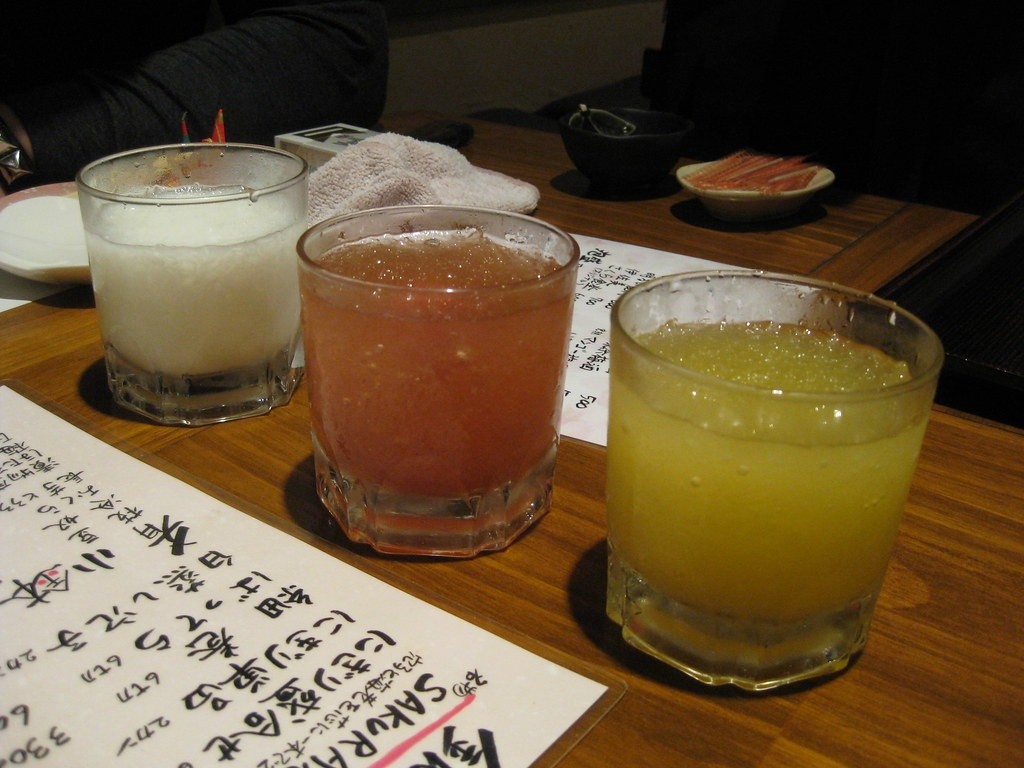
75;141;310;424
603;269;943;688
294;205;583;558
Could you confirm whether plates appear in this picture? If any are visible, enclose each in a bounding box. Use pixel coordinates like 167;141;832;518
674;160;835;219
0;194;92;287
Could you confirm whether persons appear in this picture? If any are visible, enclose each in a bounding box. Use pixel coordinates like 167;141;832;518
0;0;386;195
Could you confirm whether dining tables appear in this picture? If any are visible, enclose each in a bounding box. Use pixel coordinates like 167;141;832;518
1;172;1022;767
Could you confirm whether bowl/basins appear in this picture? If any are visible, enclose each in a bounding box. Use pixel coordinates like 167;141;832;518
556;100;695;197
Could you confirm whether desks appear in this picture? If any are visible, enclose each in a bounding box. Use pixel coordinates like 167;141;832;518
376;109;981;337
